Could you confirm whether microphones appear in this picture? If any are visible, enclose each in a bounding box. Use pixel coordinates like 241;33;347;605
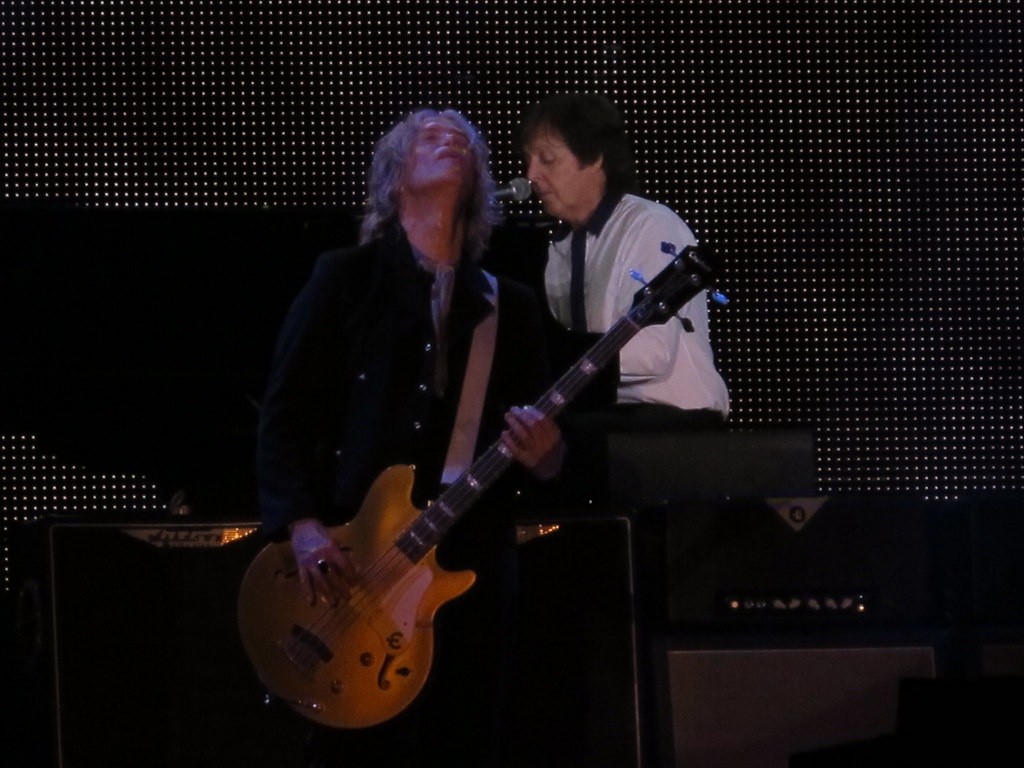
492;179;531;203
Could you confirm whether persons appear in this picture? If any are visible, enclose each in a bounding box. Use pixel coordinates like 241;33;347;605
249;109;576;768
524;95;732;414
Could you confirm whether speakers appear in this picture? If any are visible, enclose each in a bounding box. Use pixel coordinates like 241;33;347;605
48;520;270;768
497;420;1024;768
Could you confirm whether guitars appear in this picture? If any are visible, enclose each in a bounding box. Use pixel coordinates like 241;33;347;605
221;237;734;731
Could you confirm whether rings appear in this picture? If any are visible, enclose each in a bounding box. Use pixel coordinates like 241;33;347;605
318;560;328;571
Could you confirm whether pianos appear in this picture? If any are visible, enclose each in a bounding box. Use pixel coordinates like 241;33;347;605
2;206;817;639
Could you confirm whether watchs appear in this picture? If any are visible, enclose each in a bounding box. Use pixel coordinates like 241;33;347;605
536;443;565;479
